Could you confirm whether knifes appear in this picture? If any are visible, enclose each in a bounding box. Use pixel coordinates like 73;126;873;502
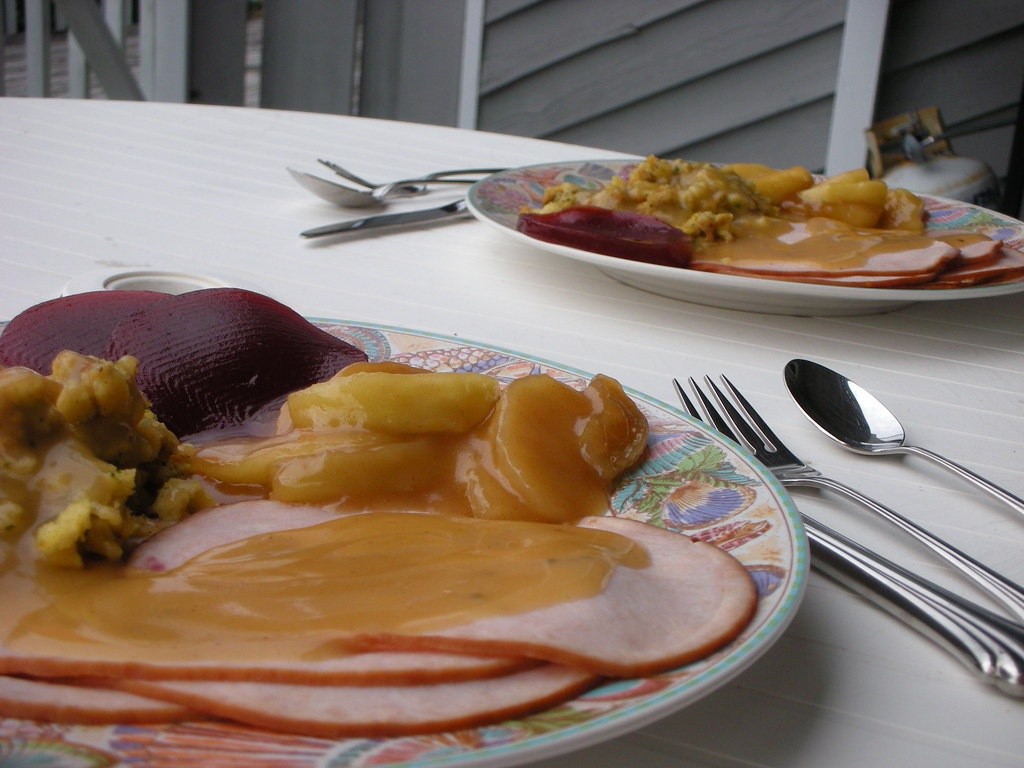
299;198;474;240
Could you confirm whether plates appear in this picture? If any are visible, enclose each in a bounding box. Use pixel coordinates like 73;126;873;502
465;159;1024;318
0;314;811;768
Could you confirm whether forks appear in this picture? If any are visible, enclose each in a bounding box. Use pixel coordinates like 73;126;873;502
318;158;504;191
672;372;1024;626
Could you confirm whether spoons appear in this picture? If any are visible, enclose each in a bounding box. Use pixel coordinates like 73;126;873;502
286;166;478;210
783;358;1024;519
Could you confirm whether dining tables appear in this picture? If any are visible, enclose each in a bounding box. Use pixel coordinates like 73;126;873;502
0;96;1024;768
